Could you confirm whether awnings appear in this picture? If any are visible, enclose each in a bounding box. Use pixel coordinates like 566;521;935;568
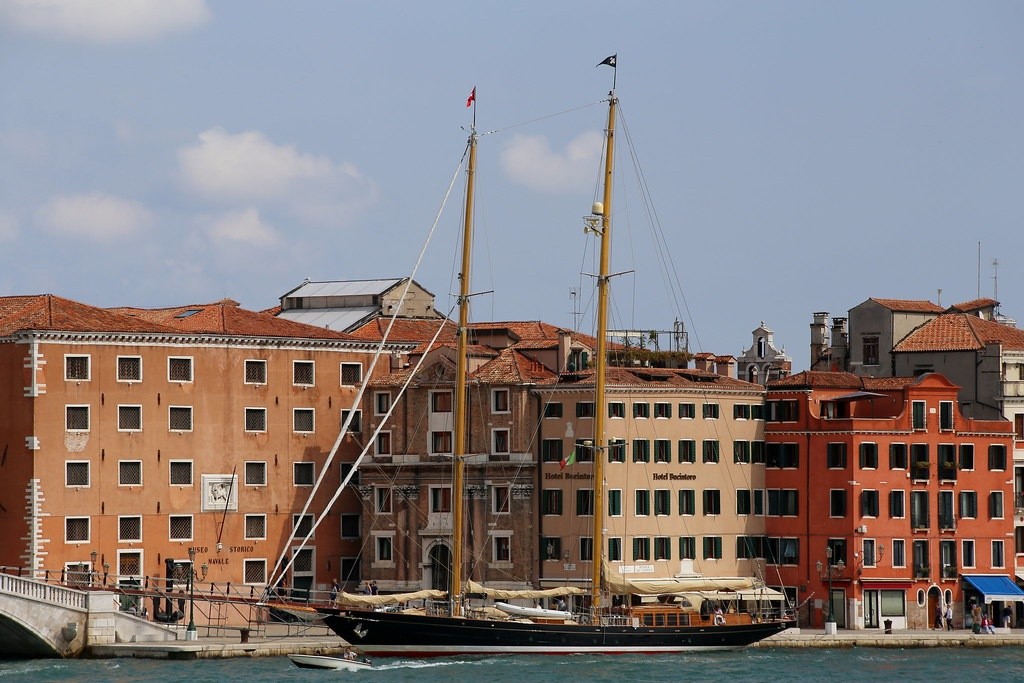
960;573;1024;604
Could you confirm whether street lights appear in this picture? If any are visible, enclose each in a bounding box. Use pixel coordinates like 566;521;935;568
177;548;209;631
816;544;846;622
91;549;109;588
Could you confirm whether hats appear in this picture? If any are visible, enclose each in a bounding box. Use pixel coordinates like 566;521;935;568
714;605;720;608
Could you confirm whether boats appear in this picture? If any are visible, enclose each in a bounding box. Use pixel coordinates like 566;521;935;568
284;651;374;672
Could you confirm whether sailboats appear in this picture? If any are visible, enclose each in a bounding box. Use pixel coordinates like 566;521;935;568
268;57;800;652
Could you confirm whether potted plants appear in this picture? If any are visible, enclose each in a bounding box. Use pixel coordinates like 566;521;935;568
918;520;927;528
919;567;931;578
945;566;958;578
941;461;961;480
943;515;949;529
608;350;695;369
916;462;932;479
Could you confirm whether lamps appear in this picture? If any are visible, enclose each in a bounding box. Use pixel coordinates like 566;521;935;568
854;544;885;576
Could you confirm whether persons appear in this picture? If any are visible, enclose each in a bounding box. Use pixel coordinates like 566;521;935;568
972;604;1011;635
365;579;379;596
128;603;137;616
141;608;149;621
934;603;944;628
330;578;340;604
943;604;955;631
